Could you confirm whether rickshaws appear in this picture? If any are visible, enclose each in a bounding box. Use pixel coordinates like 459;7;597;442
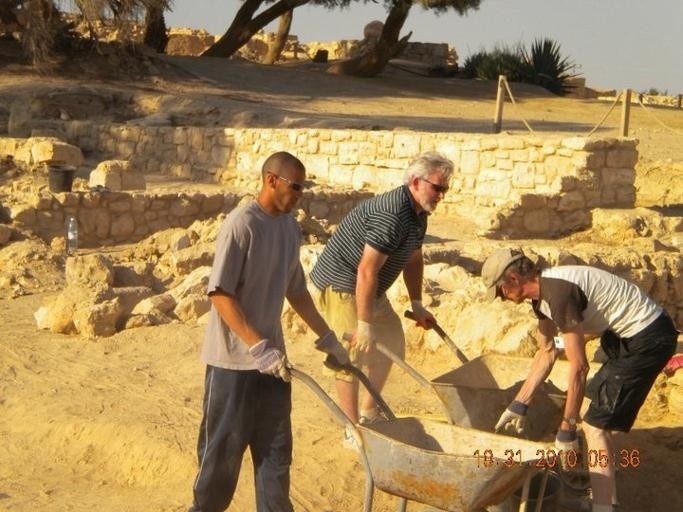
284;352;558;512
341;308;605;512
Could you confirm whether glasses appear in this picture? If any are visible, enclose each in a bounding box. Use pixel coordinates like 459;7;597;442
266;170;305;190
418;176;449;192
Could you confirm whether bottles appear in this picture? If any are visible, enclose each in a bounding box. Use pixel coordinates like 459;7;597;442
67;217;79;250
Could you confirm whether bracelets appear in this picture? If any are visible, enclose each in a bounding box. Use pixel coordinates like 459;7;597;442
555;427;578;443
507;400;529;418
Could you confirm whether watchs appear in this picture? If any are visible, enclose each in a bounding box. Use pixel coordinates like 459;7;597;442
562;417;578;426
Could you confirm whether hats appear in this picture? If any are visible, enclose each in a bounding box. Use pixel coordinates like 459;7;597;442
480;248;525;305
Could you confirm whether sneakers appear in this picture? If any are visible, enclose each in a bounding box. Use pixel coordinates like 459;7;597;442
341;414;388;452
562;493;620;512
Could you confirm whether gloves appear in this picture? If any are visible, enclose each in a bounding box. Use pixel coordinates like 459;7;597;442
313;329;352;379
494;398;527;435
353;320;377;353
249;339;294;383
411;299;435;330
555;429;581;455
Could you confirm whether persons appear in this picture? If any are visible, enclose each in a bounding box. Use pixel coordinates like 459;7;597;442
309;151;455;453
186;151;352;512
481;248;678;512
